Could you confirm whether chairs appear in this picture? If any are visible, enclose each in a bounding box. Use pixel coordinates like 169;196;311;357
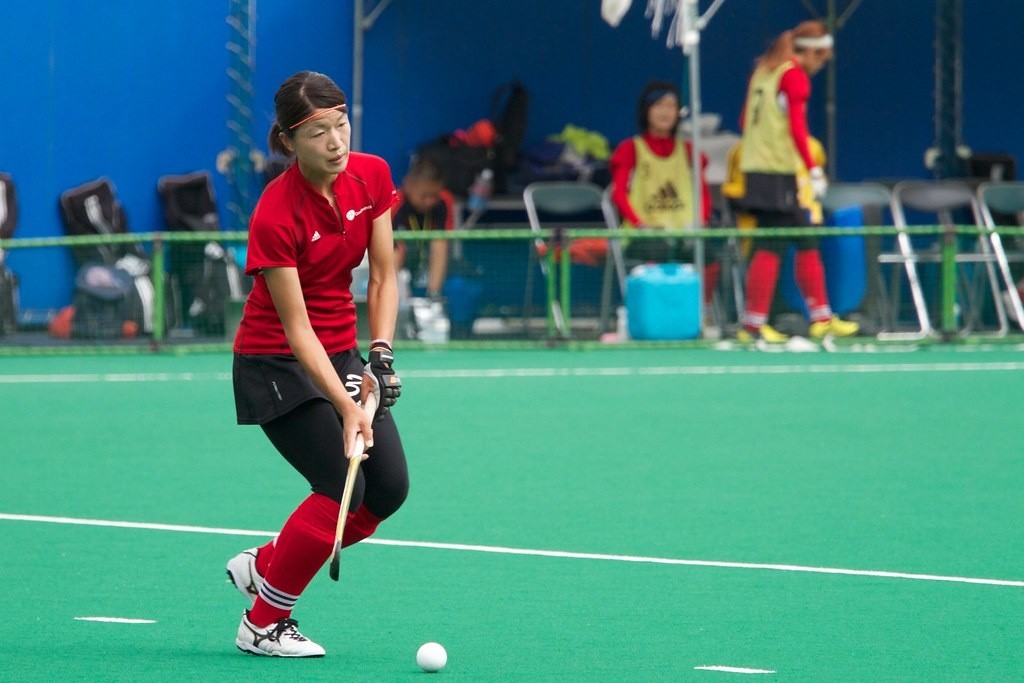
523;180;1024;339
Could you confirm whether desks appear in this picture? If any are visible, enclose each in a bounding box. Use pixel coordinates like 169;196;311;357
417;199;727;295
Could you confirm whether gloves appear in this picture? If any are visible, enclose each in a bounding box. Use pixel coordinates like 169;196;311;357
361;340;401;422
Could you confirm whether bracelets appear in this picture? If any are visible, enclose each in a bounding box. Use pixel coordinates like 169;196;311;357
369;339;392;353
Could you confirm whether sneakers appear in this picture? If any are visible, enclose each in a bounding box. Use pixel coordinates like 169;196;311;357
739;317;790;345
235;606;325;657
811;314;859;340
226;546;264;602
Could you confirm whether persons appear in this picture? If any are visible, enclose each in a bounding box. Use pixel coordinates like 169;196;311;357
226;72;409;657
390;164;455;338
724;23;858;341
610;85;719;337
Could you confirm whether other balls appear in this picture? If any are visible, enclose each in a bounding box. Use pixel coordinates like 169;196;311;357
415;641;448;673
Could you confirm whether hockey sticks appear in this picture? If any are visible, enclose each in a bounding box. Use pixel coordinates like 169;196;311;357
328;391;378;583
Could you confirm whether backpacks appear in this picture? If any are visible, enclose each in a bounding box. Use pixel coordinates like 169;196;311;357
69;266;144;340
